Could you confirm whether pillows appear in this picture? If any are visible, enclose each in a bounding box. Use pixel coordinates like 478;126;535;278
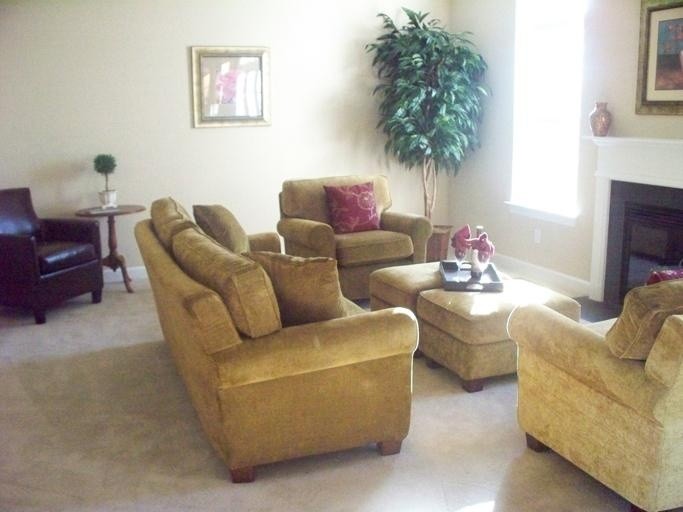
644;271;676;281
323;181;379;234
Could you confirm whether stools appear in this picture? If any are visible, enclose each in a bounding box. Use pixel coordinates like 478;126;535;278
417;279;581;393
365;263;478;360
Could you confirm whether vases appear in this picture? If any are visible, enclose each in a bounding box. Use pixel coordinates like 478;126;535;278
589;103;613;136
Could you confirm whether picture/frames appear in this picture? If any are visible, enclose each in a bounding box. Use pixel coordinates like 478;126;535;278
634;0;683;117
191;46;272;129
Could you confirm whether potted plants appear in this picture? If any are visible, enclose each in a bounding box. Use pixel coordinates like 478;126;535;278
365;7;497;261
94;155;122;210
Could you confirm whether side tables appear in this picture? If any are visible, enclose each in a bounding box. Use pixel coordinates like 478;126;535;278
75;206;141;294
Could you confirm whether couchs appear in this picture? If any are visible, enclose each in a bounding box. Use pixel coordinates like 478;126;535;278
276;174;434;302
1;188;104;324
135;197;419;485
506;276;683;507
